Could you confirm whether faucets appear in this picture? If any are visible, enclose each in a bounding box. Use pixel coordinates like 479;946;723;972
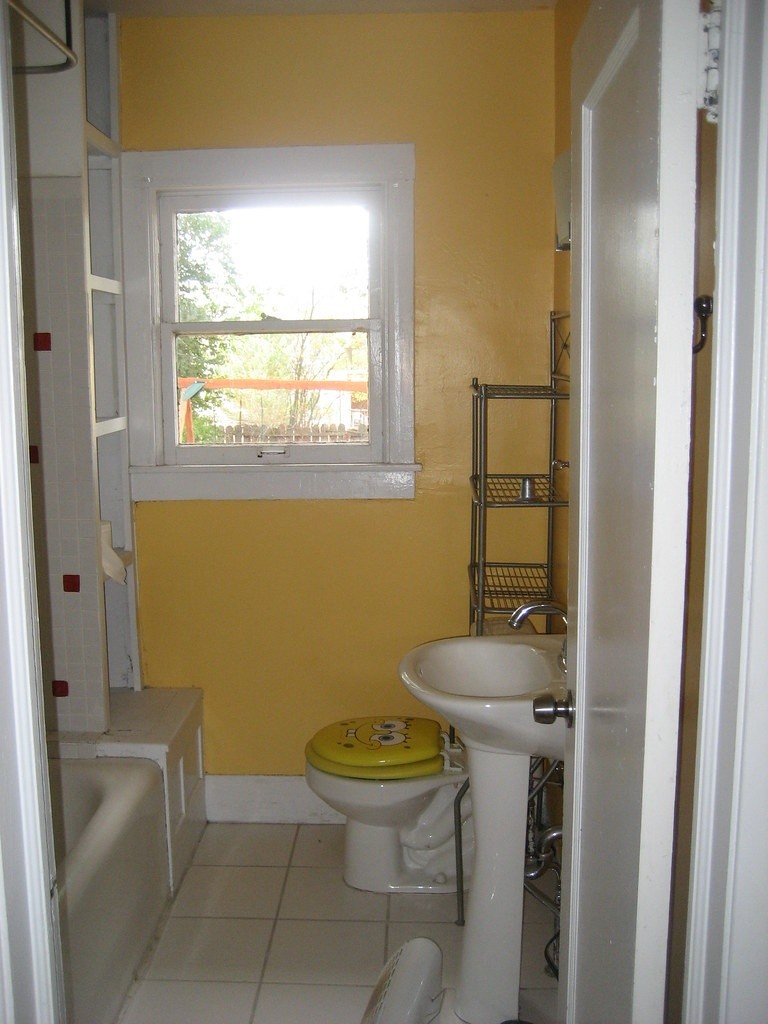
506;600;568;677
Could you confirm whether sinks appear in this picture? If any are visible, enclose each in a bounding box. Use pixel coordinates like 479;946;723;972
398;634;568;762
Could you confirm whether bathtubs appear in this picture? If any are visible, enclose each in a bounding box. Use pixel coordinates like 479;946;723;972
46;757;171;1024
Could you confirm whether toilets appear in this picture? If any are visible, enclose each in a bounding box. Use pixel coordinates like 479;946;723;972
304;614;541;894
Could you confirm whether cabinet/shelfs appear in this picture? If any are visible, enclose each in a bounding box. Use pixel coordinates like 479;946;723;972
450;310;573;744
18;0;144;733
